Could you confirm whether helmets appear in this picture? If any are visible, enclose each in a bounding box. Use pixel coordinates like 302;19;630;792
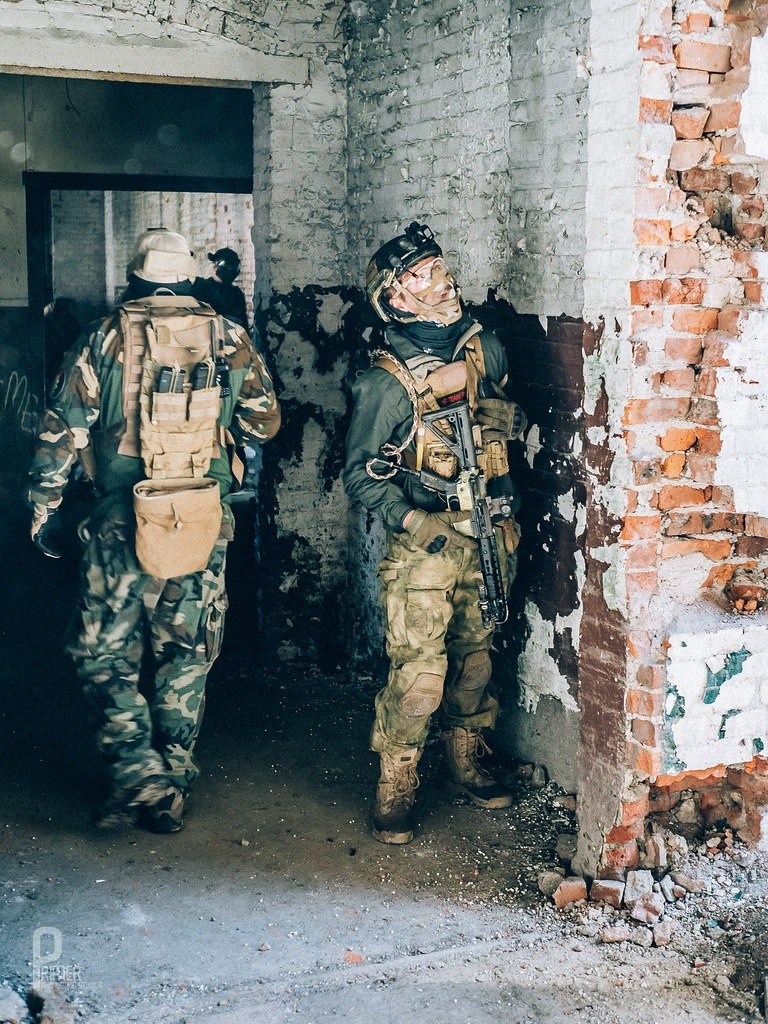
362;234;444;323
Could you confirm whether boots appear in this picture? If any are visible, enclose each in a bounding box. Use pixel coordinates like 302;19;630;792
439;723;516;811
371;747;426;848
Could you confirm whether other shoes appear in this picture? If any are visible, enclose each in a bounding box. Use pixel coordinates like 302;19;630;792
143;785;185;834
93;774;171;838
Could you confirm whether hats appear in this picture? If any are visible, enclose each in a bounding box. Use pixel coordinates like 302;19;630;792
208;246;242;272
128;226;204;288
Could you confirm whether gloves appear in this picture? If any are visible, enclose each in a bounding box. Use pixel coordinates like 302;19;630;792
405;506;483;571
477;382;530;445
24;498;70;562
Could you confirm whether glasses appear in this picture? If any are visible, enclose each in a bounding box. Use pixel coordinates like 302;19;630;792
391;257;449;299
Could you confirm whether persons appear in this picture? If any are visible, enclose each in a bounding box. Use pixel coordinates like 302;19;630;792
344;220;528;844
194;246;250;338
44;295;81;408
26;228;282;834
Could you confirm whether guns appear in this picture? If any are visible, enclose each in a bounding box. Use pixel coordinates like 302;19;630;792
375;397;511;635
